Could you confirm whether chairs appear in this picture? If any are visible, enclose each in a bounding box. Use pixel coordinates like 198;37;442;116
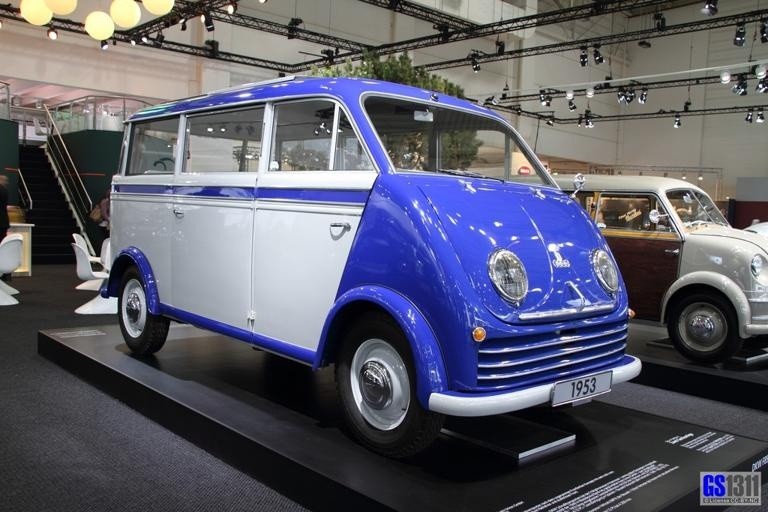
71;232;118;314
0;233;24;306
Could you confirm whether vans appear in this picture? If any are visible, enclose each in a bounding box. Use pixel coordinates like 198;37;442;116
491;173;767;367
97;71;645;465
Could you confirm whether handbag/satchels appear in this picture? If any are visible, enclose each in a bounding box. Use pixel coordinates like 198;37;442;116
89;207;103;223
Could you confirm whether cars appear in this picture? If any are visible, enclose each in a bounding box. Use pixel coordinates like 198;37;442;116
741;222;767;237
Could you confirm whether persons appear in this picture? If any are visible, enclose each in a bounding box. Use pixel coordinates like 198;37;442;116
95;188;110;250
0;174;12;284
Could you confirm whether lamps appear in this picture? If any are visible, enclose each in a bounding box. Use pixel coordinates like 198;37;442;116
47;1;238;52
